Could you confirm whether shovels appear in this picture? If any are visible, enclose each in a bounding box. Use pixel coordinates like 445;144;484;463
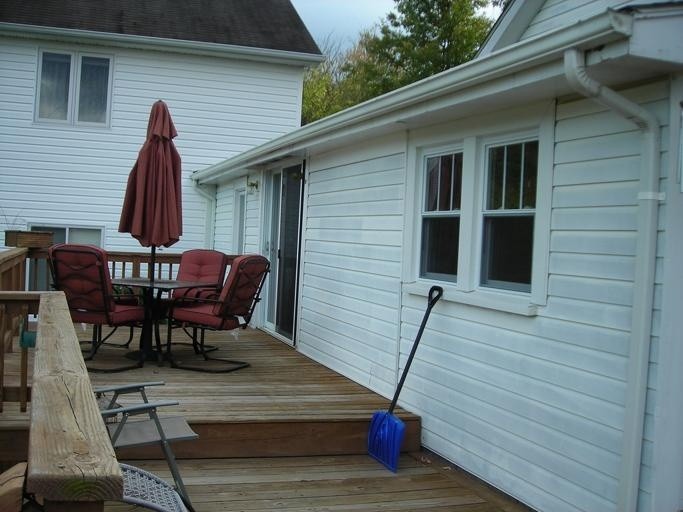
368;286;443;473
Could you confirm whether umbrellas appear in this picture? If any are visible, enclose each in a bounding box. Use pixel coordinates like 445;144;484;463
117;100;183;282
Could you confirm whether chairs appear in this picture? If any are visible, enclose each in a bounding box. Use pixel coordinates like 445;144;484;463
44;244;272;376
88;379;200;512
